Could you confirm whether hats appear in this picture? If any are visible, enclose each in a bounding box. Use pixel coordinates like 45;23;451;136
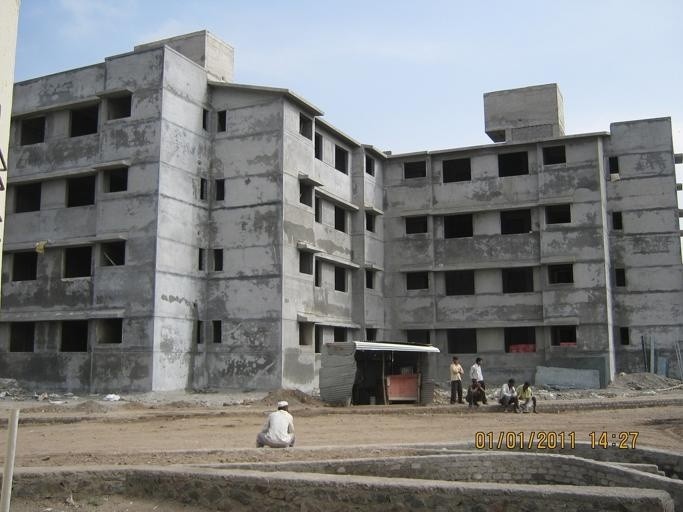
278;401;288;407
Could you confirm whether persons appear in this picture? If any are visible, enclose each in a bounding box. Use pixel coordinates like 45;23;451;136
515;381;538;413
469;358;488;406
465;378;488;408
497;378;521;413
449;356;465;405
256;400;295;448
